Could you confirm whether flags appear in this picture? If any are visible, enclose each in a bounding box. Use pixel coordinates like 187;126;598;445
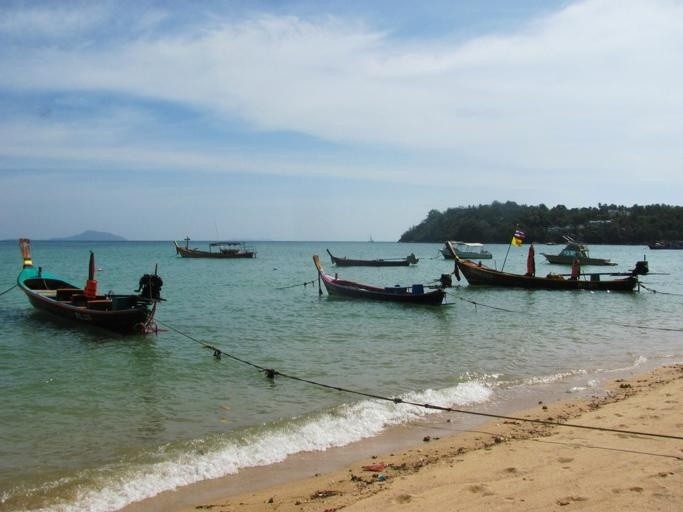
510;229;526;248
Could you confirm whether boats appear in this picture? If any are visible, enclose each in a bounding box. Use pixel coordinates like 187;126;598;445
18;239;166;335
173;236;257;258
648;238;683;249
